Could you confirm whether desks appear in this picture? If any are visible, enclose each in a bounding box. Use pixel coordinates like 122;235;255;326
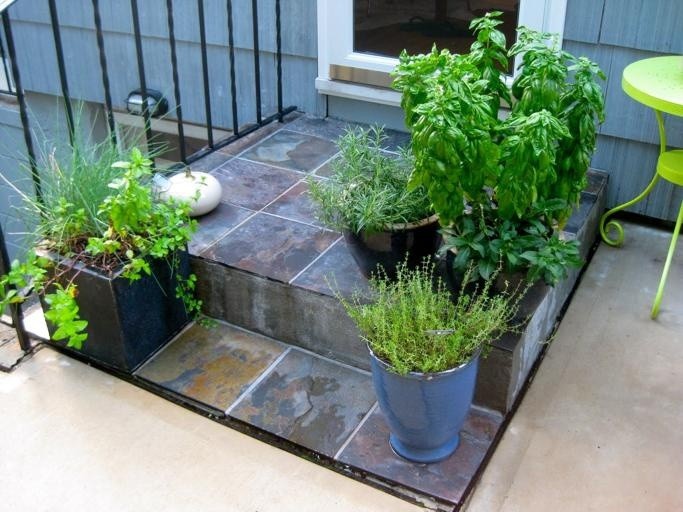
599;54;682;319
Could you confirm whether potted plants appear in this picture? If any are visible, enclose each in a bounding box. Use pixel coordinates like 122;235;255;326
299;121;446;286
322;256;559;466
1;79;219;376
386;6;607;319
